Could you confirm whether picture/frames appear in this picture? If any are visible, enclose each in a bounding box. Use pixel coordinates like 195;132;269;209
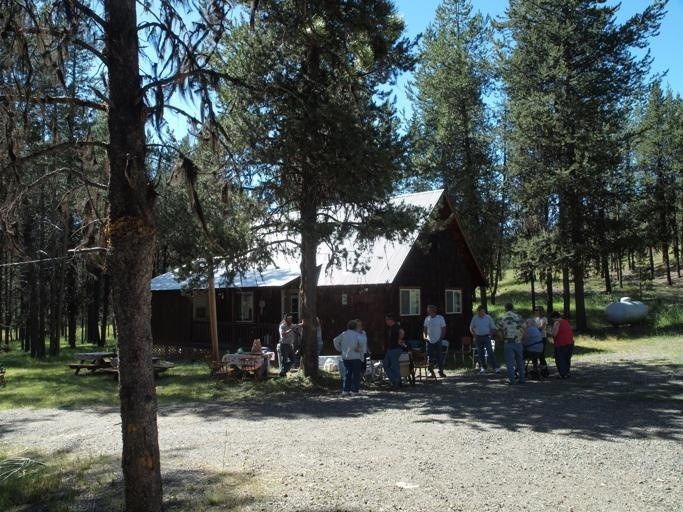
221;352;275;378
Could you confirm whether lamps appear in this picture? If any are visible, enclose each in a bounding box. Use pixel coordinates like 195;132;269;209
211;359;257;384
385;337;550;383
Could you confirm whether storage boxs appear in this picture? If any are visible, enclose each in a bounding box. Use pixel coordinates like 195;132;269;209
63;350;174;381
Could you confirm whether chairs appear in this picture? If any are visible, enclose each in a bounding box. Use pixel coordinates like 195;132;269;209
342;391;359;396
428;371;446;378
386;384;402;390
480;366;572;385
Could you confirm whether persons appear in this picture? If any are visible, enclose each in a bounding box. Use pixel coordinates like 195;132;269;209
378;312;406;390
353;318;368;384
469;304;501;374
422;302;449;378
531;305;548;377
333;319;369;395
496;303;530;385
275;313;324;377
519;319;543;360
550;310;574;378
